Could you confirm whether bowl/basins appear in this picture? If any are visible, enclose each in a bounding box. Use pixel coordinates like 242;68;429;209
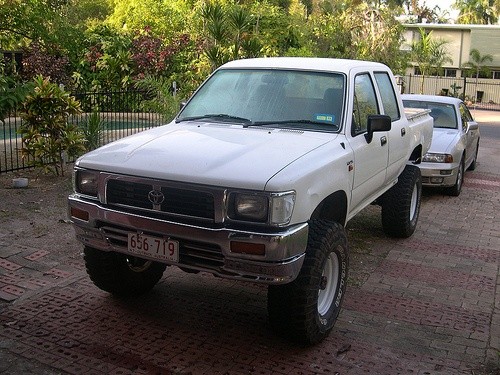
12;178;28;188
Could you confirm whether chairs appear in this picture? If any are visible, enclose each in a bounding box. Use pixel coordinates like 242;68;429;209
325;88;344;125
434;107;455;127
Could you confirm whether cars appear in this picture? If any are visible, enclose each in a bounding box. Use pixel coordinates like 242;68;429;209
400;93;480;194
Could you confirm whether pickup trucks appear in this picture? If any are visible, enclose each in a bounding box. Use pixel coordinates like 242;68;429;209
67;58;434;342
